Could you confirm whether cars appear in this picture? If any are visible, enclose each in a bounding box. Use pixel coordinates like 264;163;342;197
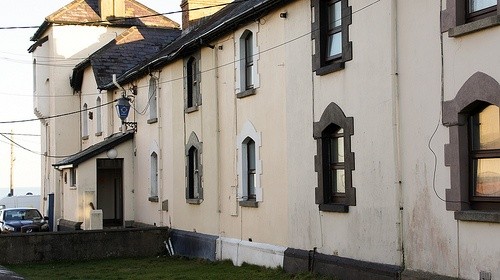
0;208;51;234
